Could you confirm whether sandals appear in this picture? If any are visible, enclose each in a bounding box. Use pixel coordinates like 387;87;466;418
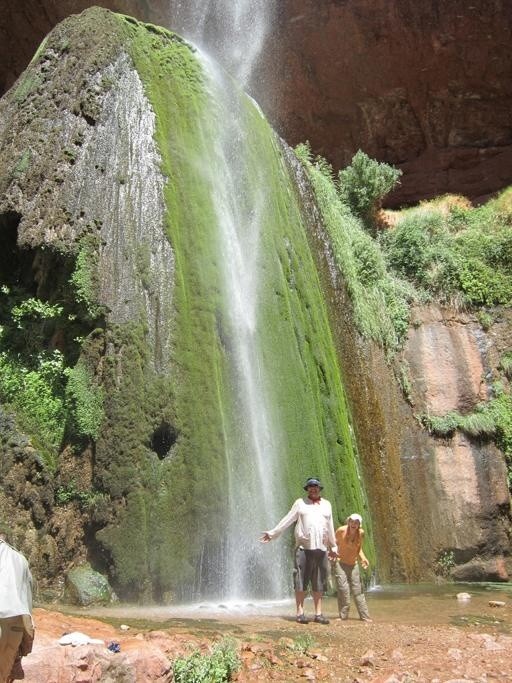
296;614;308;624
315;615;329;624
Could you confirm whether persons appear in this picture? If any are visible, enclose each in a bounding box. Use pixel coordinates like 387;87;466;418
328;514;372;623
0;539;35;683
258;477;341;624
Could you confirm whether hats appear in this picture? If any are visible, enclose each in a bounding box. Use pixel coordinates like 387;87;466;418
302;478;324;491
346;513;363;528
